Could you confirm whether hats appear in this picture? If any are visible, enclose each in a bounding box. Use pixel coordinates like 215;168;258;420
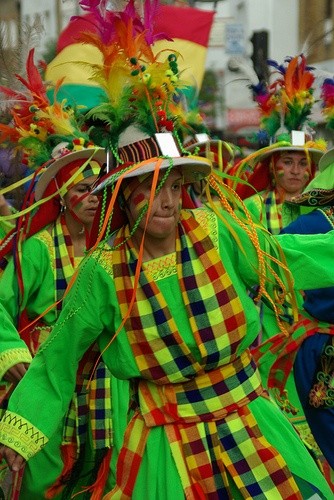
183;133;232;166
35;142;113;202
259;125;324;165
90;121;211;194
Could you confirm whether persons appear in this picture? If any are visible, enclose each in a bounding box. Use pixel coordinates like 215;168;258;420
0;141;129;500
229;127;325;237
0;121;334;500
182;133;244;204
280;148;334;472
